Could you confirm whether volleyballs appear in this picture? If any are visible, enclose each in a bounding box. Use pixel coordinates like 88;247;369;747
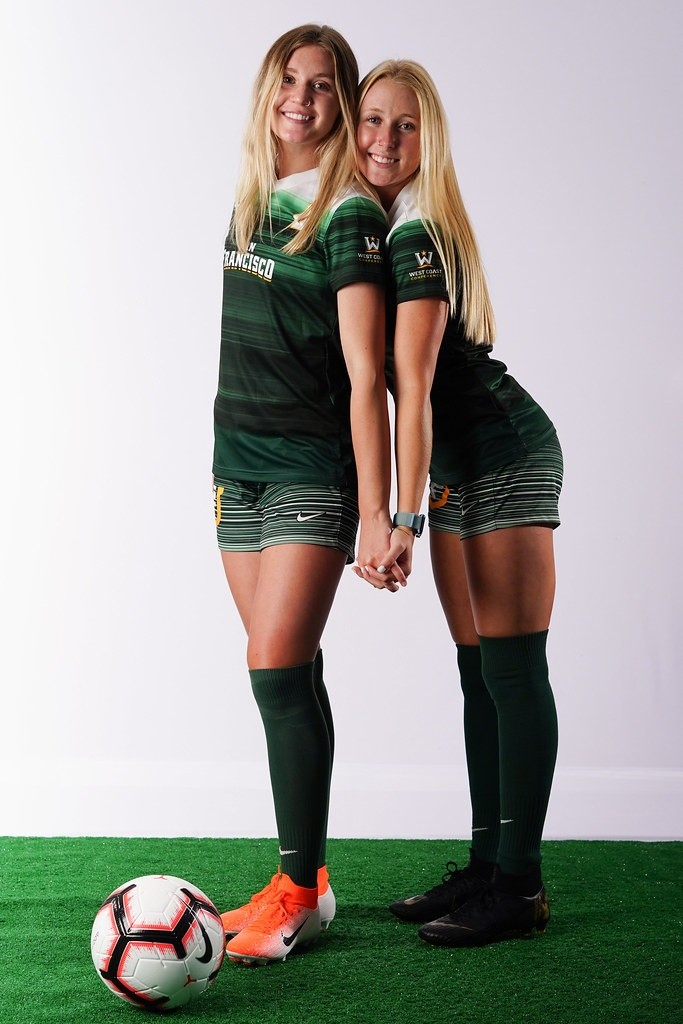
90;875;223;1010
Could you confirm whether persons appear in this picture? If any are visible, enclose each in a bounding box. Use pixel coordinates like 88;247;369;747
356;58;563;948
207;23;404;964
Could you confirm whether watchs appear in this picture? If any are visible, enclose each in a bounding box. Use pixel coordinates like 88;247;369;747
392;512;426;538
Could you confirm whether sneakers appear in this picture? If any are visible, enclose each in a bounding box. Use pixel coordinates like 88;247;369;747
389;867;550;948
219;873;335;965
